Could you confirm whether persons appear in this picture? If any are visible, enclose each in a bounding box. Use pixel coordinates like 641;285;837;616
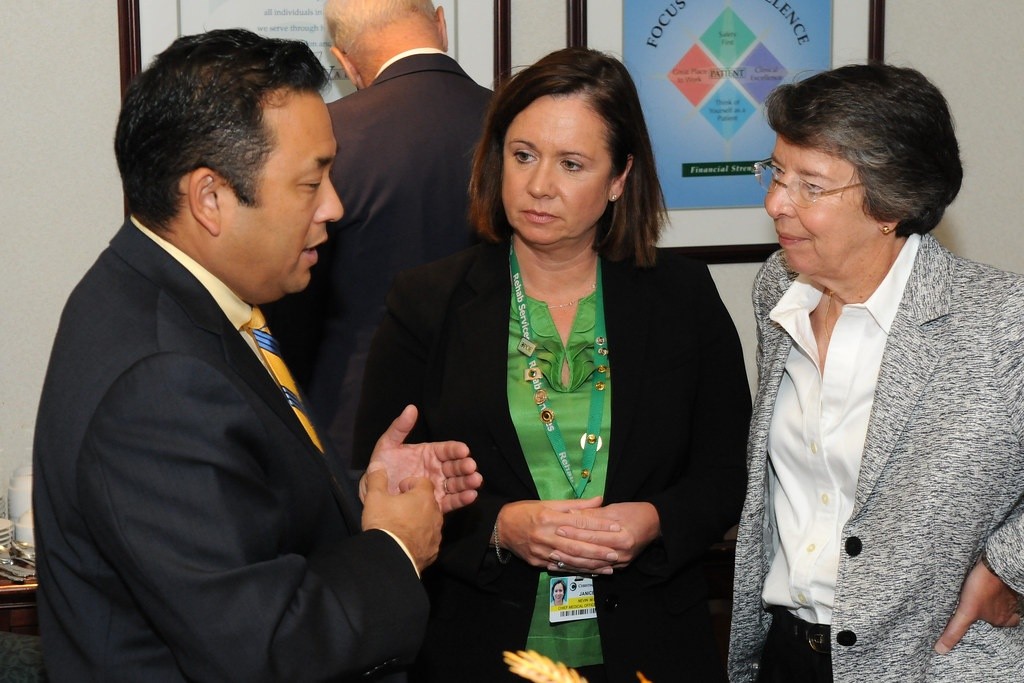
728;65;1024;683
33;29;484;683
552;581;566;605
355;46;754;683
267;1;494;473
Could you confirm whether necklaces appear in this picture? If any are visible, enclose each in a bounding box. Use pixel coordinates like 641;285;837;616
825;292;831;343
549;285;596;309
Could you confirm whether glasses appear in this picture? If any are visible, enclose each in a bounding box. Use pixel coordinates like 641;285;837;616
753;160;866;209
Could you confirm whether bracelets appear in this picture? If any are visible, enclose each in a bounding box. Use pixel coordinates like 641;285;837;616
494;521;510;563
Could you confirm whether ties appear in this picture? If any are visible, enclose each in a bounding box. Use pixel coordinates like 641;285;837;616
240;302;325;458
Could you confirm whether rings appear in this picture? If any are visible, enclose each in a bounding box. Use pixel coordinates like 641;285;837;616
558;562;564;567
445;479;449;493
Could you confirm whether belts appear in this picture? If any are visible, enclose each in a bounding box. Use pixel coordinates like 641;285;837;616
775;606;830;655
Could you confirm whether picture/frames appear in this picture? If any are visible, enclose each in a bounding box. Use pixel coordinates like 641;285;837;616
117;0;511;111
568;1;885;264
